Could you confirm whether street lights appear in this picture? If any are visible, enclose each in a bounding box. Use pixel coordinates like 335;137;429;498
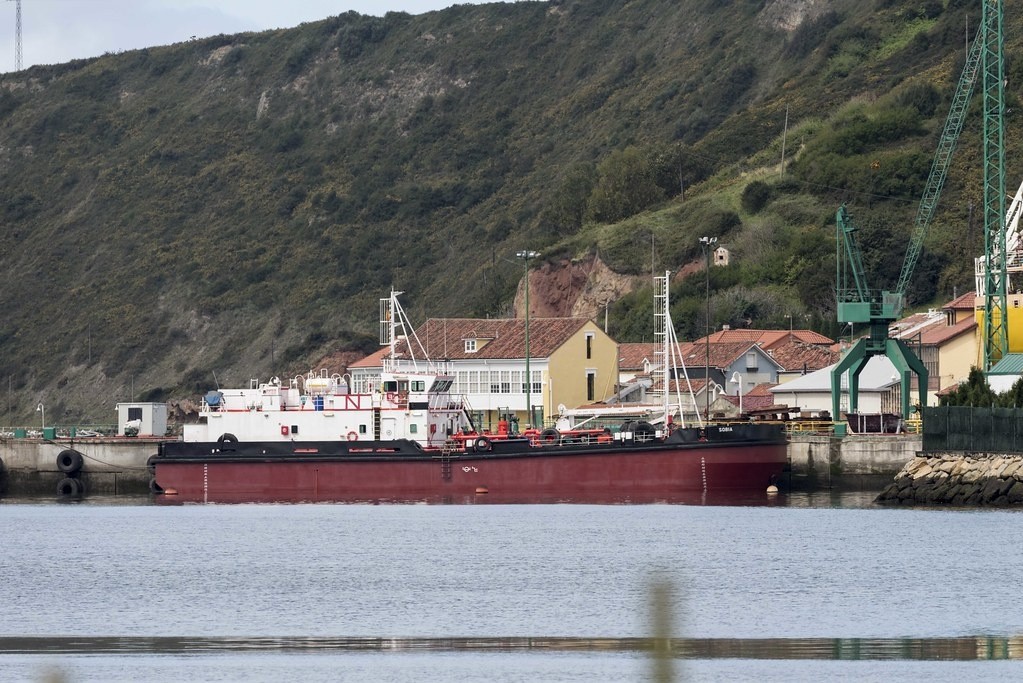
698;237;718;424
516;250;542;430
37;401;45;429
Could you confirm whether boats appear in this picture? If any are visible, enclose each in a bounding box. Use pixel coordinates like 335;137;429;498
145;271;789;507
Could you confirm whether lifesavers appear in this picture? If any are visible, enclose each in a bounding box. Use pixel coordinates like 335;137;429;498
218;432;238;442
347;431;358;442
578;433;592;446
56;450;87;498
392;394;403;404
620;423;654;447
475;436;491;452
148;454;163;493
540;428;560;447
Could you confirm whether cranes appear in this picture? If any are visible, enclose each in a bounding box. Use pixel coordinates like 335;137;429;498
830;0;1011;439
